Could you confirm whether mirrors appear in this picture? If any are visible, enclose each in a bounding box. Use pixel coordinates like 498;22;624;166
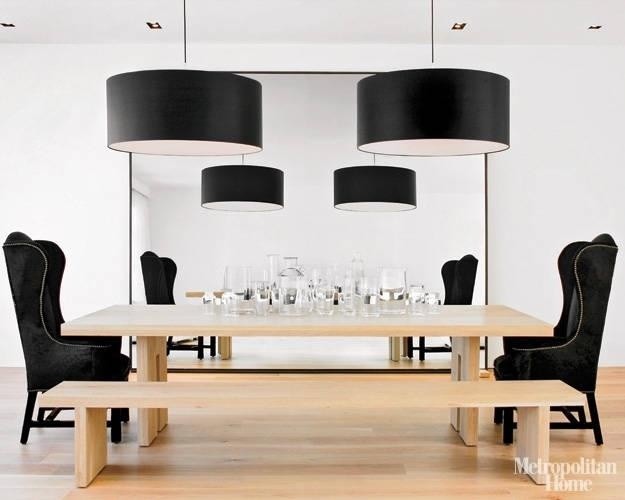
128;70;489;375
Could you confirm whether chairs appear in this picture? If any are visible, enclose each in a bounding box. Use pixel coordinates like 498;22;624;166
493;232;619;446
1;229;130;445
138;251;215;358
406;254;480;361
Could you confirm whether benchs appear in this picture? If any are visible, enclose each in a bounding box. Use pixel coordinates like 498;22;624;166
38;379;587;489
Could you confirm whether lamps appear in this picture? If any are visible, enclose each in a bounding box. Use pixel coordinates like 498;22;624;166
200;152;417;213
105;1;510;156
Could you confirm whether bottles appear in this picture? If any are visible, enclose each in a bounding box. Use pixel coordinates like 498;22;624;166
203;253;441;317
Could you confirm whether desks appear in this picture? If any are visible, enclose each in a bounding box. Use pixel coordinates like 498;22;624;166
183;288;409;362
60;305;554;448
